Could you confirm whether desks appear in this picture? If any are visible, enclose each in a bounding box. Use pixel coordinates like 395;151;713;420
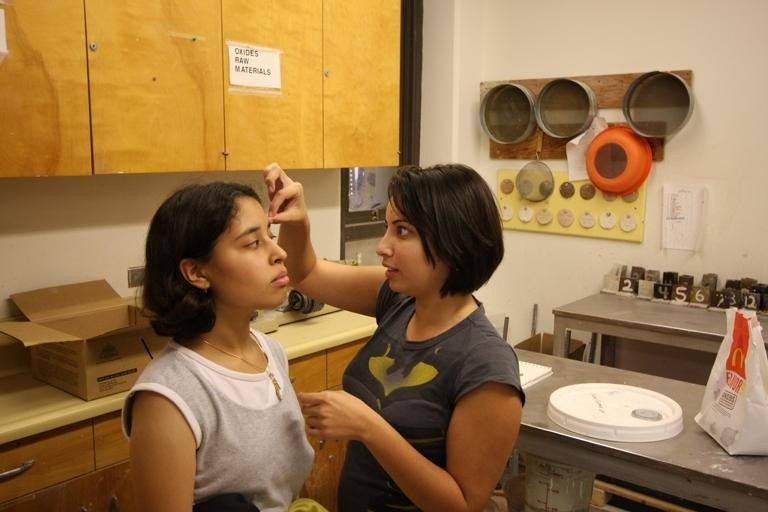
545;289;767;364
503;345;767;511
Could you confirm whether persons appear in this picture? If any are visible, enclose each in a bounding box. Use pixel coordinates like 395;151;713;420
262;161;525;512
120;181;315;510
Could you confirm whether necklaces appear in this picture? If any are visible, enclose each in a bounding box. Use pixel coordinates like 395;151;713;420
202;330;282;402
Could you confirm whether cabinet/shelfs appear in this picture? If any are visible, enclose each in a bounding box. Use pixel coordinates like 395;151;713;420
219;1;401;173
1;407;136;512
285;336;372;512
1;0;225;179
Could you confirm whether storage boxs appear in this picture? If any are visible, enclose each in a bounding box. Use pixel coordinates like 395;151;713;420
511;332;586;363
0;277;174;404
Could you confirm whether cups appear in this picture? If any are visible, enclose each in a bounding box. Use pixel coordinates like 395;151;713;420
604;263;767;314
